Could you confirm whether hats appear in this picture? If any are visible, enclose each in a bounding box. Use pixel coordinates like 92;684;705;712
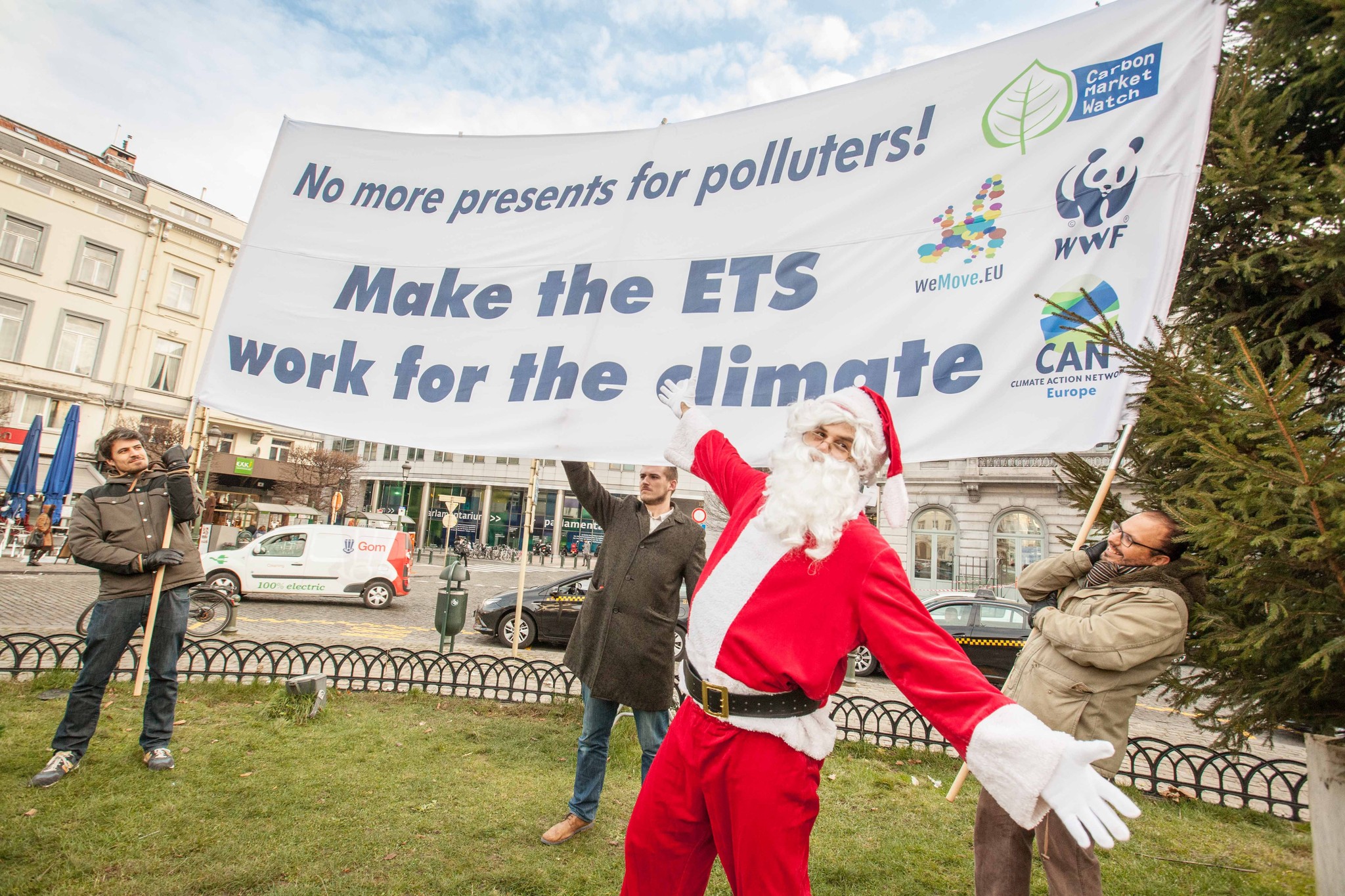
830;378;910;530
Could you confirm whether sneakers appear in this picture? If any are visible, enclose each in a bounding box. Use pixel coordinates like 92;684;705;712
143;747;174;771
29;751;81;788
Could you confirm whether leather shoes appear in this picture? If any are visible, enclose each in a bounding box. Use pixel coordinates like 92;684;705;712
541;813;594;845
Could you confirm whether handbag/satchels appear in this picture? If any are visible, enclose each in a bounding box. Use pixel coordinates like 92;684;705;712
463;548;469;554
585;554;591;560
29;529;45;548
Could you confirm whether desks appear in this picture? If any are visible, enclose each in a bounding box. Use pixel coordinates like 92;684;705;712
0;522;71;559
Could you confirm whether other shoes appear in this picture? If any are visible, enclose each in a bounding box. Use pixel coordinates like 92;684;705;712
582;564;584;566
464;566;468;567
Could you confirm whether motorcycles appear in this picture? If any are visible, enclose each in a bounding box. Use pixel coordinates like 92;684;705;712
571;541;579;557
561;544;568;557
529;540;552;557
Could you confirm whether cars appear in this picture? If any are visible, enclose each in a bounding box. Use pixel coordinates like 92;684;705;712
475;570;696;663
843;589;1043;693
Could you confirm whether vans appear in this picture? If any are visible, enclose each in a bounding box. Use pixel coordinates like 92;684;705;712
198;524;415;611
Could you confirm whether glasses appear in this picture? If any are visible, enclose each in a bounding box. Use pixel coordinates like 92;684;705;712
802;430;853;463
1110;519;1168;556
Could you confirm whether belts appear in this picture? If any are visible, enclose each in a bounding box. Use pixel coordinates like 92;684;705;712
683;659;822;720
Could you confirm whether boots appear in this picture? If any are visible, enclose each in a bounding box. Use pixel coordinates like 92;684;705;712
30;551;45;566
27;551;36;566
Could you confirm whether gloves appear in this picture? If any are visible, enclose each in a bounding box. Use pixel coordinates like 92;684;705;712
161;443;194;472
657;375;698;417
1027;590;1058;629
1080;540;1108;566
1039;739;1142;851
141;548;185;573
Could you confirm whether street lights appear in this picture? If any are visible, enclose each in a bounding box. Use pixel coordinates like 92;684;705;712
397;460;411;530
190;425;222;552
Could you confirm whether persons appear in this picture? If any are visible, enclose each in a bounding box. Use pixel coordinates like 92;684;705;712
458;538;468;568
253;525;267;539
974;512;1191;896
294;538;305;557
25;503;56;566
582;538;590;566
32;425;205;789
246;521;257;536
615;375;1141;896
541;459;707;849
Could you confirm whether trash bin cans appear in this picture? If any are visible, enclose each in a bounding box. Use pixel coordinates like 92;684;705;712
446;553;457;567
434;560;471;657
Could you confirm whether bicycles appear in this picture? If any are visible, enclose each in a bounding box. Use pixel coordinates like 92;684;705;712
76;584;236;640
455;544;521;563
